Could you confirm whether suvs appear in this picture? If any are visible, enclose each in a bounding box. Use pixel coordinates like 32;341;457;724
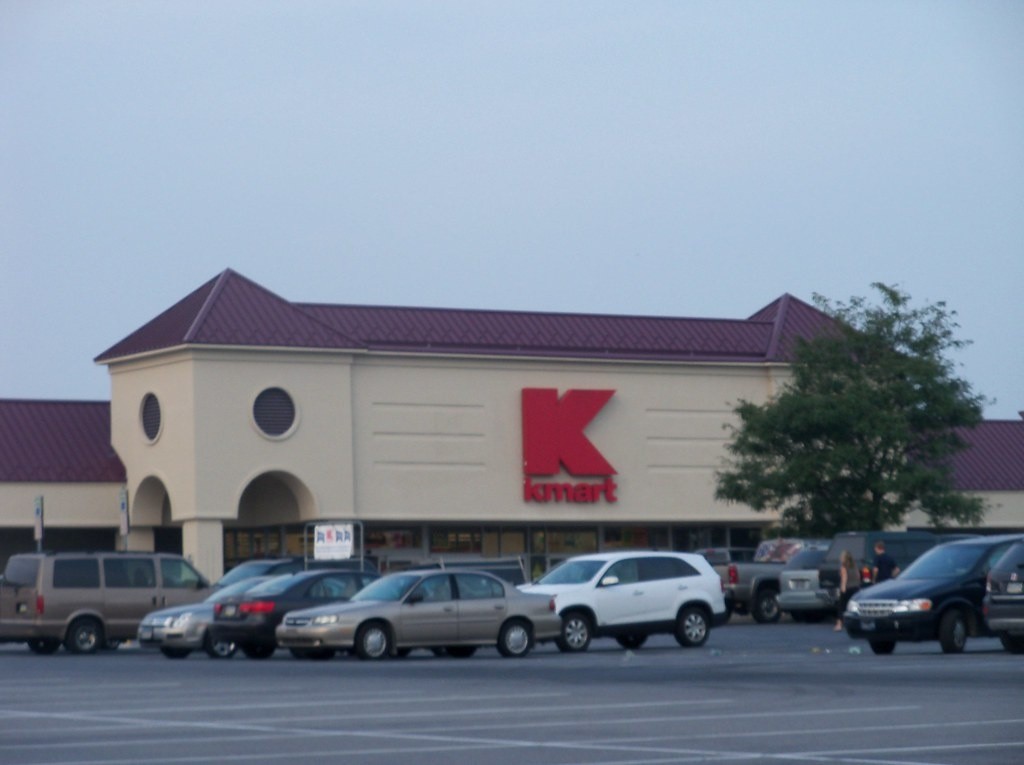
982;538;1024;655
213;557;383;588
817;527;984;601
0;550;240;660
695;547;756;567
774;532;844;622
515;550;729;654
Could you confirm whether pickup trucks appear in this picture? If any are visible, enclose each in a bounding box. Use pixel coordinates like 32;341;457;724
709;537;816;622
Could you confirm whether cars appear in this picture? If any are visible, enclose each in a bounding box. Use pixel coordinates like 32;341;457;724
839;533;1023;653
211;567;421;662
136;576;351;663
273;572;562;661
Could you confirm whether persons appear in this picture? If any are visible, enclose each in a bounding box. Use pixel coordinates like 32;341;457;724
833;550;860;631
872;540;900;584
